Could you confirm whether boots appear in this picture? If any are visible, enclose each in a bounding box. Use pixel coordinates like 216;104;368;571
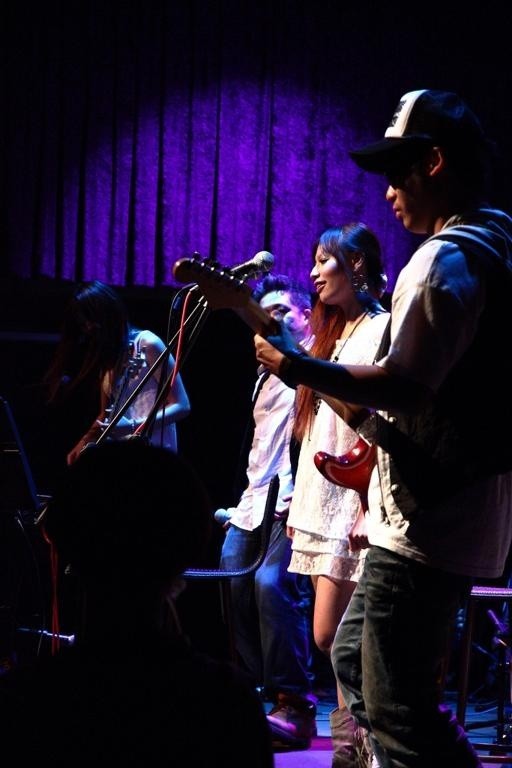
330;708;381;768
264;692;316;749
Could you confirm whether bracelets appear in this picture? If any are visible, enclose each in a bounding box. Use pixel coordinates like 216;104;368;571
276;346;310;391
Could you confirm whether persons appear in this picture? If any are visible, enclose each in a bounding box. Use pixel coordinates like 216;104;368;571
1;437;275;767
282;220;395;768
215;270;320;753
252;87;511;768
60;275;192;468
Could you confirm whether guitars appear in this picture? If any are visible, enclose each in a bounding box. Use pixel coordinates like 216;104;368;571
172;250;379;516
94;346;146;439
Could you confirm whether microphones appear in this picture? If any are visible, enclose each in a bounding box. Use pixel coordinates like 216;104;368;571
188;250;275;293
214;508;231;525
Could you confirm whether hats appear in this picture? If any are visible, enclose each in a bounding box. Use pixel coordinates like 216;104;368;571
348;90;485;173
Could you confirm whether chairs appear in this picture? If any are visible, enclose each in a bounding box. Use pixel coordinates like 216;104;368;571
177;474;279;673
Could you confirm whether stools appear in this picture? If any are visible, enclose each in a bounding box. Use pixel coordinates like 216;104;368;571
457;585;512;745
